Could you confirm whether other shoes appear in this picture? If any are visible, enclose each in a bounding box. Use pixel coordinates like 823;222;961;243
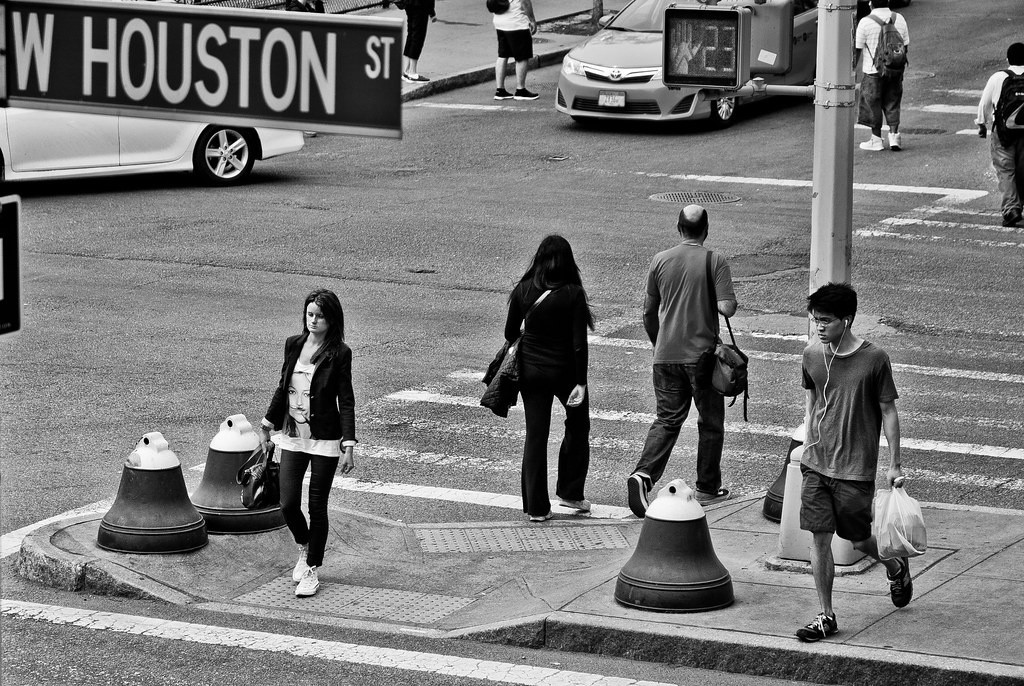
1001;208;1022;226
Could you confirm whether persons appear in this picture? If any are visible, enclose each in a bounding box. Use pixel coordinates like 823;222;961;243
258;288;357;595
795;283;912;643
978;43;1024;225
492;0;539;101
402;0;437;83
856;0;910;151
505;236;594;522
628;204;739;518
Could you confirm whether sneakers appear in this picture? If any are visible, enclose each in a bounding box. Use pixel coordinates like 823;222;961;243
796;612;839;643
530;510;553;521
886;556;913;608
888;132;902;151
292;542;309;582
401;74;430;84
859;135;884;151
295;566;320;597
494;89;514;100
695;488;730;506
514;88;540;101
627;474;649;518
559;498;591;511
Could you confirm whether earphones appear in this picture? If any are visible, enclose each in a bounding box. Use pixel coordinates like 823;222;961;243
845;320;849;327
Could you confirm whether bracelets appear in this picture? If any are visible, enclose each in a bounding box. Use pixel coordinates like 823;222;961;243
261;423;271;432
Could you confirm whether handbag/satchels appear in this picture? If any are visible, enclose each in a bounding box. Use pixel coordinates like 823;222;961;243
236;440;280;510
711;344;748;397
873;475;927;561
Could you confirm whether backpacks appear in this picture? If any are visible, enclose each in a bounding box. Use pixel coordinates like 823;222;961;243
394;0;408;10
991;70;1024;149
486;0;510;14
865;11;907;84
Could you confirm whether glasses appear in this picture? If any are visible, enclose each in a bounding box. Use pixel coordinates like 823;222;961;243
810;317;838;327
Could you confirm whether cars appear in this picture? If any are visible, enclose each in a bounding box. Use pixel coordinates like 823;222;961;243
548;0;864;127
0;93;305;182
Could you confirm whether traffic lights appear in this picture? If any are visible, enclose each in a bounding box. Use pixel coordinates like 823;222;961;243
664;7;753;89
